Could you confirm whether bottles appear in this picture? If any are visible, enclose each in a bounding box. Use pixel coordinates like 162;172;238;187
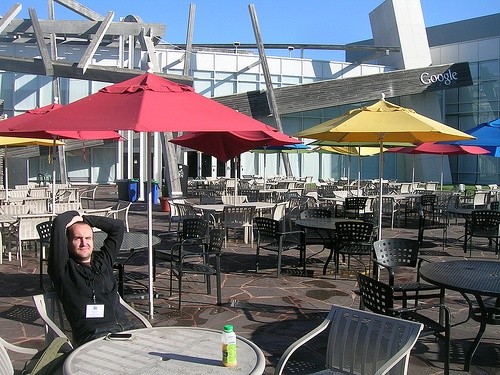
222;325;237;367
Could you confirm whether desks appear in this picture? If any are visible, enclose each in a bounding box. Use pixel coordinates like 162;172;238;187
446;208;487;251
64;325;267;375
195;201;276;245
319;197;346;219
0;219;18;265
376;194;426;227
91;232;161;300
417;260;500;371
294;218;369;275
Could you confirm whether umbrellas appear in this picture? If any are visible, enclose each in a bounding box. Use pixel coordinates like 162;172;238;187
0;72;279;320
0;103;126;215
168;93;500;281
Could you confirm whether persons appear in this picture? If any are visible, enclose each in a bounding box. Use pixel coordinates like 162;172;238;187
47;210;140;348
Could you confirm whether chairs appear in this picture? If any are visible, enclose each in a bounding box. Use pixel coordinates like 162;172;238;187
0;173;500;375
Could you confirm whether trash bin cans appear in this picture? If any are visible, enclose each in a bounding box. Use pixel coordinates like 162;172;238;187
158;197;170;212
115;178;140;203
143;180;161;205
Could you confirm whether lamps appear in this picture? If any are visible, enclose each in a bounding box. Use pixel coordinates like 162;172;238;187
233;42;240;53
288;46;294;57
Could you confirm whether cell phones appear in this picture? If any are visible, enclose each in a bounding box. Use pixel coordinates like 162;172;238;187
109;334;132;341
